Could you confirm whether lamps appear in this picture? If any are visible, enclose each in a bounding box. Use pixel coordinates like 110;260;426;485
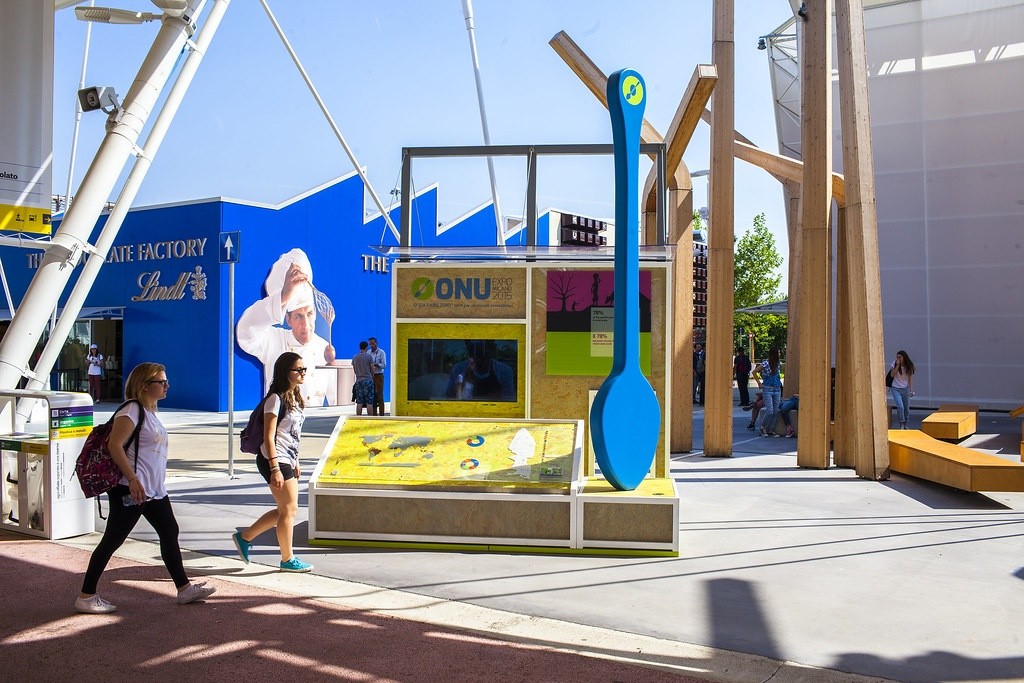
75;7;162;23
798;3;806;16
758;33;797;50
76;85;123;121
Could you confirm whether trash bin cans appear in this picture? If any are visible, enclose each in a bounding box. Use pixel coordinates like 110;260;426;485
0;390;94;540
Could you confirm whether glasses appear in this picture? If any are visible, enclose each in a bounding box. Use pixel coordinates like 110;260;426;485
290;367;307;376
148;378;168;387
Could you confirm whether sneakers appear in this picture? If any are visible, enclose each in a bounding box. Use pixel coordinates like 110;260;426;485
743;403;755;411
75;594;117;613
280;557;314;572
746;424;755;429
759;427;769;436
786;427;796;437
233;532;253;564
178;583;217;603
768;431;780;437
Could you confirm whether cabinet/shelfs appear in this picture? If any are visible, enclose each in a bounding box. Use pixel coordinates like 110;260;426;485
101;369;121;399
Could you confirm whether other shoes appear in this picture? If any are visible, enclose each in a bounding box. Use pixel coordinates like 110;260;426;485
692;400;700;404
95;399;100;404
738;402;750;406
899;424;910;430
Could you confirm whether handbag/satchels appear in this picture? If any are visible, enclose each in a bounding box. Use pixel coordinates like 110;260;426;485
885;360;898;387
100;366;105;379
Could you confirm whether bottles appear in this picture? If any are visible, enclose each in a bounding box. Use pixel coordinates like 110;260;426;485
121;489;160;506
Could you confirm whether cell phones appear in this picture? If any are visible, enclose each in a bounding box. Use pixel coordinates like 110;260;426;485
122;494;139;507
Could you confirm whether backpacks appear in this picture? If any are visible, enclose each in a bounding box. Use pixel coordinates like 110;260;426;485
240;391;285;454
74;399;145;499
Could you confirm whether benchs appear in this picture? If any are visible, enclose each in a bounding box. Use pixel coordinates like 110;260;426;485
760;403;1024;492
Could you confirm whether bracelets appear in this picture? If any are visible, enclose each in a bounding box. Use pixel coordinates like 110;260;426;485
128;476;138;481
910;389;912;390
269;465;280;473
268;456;277;461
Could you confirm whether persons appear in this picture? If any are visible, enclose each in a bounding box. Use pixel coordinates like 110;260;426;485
752;346;782;437
85;344;103;403
74;362;216;615
779;394;799;437
366;337;387;416
742;382;783;430
891;350;915;430
732;346;751;406
236;247;331;407
447;338;515;401
693;343;706;406
352;341;374;415
233;352;313;572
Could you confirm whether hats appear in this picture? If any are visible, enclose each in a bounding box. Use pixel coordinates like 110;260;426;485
90;344;98;348
264;249;314;312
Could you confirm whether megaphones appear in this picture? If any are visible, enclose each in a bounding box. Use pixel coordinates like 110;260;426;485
77;86;117;112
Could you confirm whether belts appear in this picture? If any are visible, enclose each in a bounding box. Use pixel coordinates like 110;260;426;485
375;373;383;375
